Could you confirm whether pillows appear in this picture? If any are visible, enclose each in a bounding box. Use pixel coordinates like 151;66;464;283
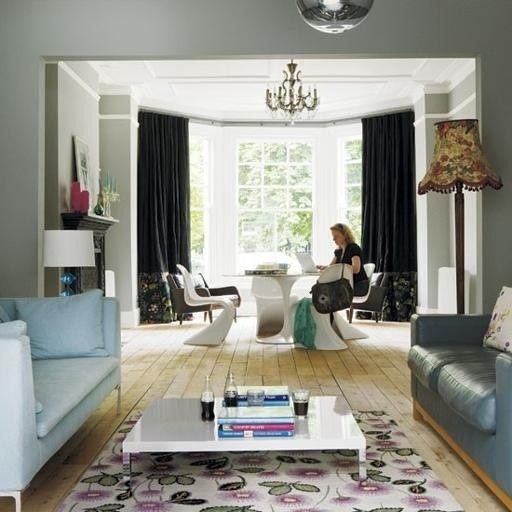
15;289;109;357
485;283;512;350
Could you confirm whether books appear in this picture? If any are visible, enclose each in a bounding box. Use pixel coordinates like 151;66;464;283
216;385;295;438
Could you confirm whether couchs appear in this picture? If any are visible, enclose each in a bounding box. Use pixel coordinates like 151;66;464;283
0;292;122;511
406;286;511;505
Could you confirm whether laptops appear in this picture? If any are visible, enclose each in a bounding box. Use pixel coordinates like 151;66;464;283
294;253;323;273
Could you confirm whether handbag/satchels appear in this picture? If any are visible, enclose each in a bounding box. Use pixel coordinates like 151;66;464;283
309;278;354;314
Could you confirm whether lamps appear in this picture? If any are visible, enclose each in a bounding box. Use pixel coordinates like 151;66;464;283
266;57;319;124
43;230;96;296
416;118;503;312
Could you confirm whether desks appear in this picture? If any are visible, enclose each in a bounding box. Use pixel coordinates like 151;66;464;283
245;269;326;344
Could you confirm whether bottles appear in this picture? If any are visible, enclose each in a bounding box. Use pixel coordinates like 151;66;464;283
223;370;239;408
198;373;217;422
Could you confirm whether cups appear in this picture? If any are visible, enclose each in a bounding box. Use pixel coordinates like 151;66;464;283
292;390;310;417
247;389;267;407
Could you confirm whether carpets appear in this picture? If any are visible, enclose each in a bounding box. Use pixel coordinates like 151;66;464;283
57;404;463;511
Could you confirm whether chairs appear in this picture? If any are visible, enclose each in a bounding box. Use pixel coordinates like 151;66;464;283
252;263;298;343
165;273;240;327
299;262;391;350
174;264;236;346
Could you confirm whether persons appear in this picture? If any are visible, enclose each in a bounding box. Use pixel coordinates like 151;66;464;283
315;222;371;325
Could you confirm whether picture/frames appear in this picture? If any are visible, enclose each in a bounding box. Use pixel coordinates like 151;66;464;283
72;135;97;214
